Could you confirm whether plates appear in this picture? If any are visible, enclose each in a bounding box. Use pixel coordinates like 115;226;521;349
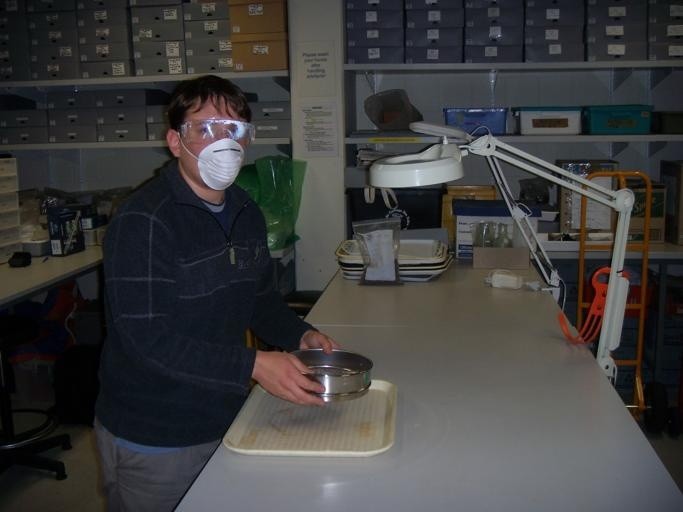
335;240;454;282
222;379;396;456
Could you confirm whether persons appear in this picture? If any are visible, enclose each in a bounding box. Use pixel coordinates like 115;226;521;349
92;75;339;511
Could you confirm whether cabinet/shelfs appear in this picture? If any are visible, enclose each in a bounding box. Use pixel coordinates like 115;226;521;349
0;135;292;155
343;130;683;147
0;68;292;89
345;54;683;72
364;227;678;270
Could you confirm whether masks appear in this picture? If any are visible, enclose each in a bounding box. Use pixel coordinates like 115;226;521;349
180;138;246;191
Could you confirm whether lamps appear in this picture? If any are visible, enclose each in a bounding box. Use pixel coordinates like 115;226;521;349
411;122;563;304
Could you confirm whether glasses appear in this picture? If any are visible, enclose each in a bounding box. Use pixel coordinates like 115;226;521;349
180;118;256;144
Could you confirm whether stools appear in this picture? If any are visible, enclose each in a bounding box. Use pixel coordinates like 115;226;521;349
1;300;75;481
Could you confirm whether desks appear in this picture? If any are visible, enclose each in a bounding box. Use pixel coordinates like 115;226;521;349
0;244;105;312
172;253;682;512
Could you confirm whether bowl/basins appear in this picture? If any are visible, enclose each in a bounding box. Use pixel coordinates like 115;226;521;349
289;349;374;402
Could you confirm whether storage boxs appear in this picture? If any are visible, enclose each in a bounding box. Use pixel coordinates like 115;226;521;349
0;245;23;264
99;121;142;141
452;198;533;262
465;25;525;48
616;178;667;243
149;122;174;140
77;1;130;12
248;119;294;137
585;41;646;62
649;14;661;26
183;38;233;58
228;2;288;33
405;49;463;64
346;47;405;64
1;52;29;66
1;177;21;194
47;107;98;125
82;61;134;77
241;102;293;120
32;47;83;65
1;16;29;34
0;194;18;214
1;227;23;248
132;41;187;58
27;30;81;48
29;2;77;14
126;2;179;7
583;104;655;135
346;14;405;30
591;2;650;20
511;105;583;135
463;46;526;65
131;26;186;44
648;2;662;15
185;55;234;73
441;106;507;135
369;134;636;386
346;30;406;49
648;26;661;43
466;10;525;28
1;38;32;50
238;90;259;104
586;23;647;42
134;59;184;77
183;5;230;19
230;34;289;72
27;14;81;31
3;126;51;146
1;2;26;16
526;0;586;8
443;185;496;247
10;106;51;129
74;11;130;26
1;158;19;177
99;86;169;110
99;105;146;125
77;48;134;62
10;66;32;81
346;186;443;229
54;87;93;108
1;210;20;231
525;26;583;43
647;41;662;61
524;8;586;27
75;28;131;44
347;0;406;11
406;2;467;12
128;8;185;22
183;21;232;38
32;62;76;79
146;104;178;122
49;124;95;143
466;1;526;9
556;158;618;238
526;43;585;62
406;7;466;30
406;27;464;48
